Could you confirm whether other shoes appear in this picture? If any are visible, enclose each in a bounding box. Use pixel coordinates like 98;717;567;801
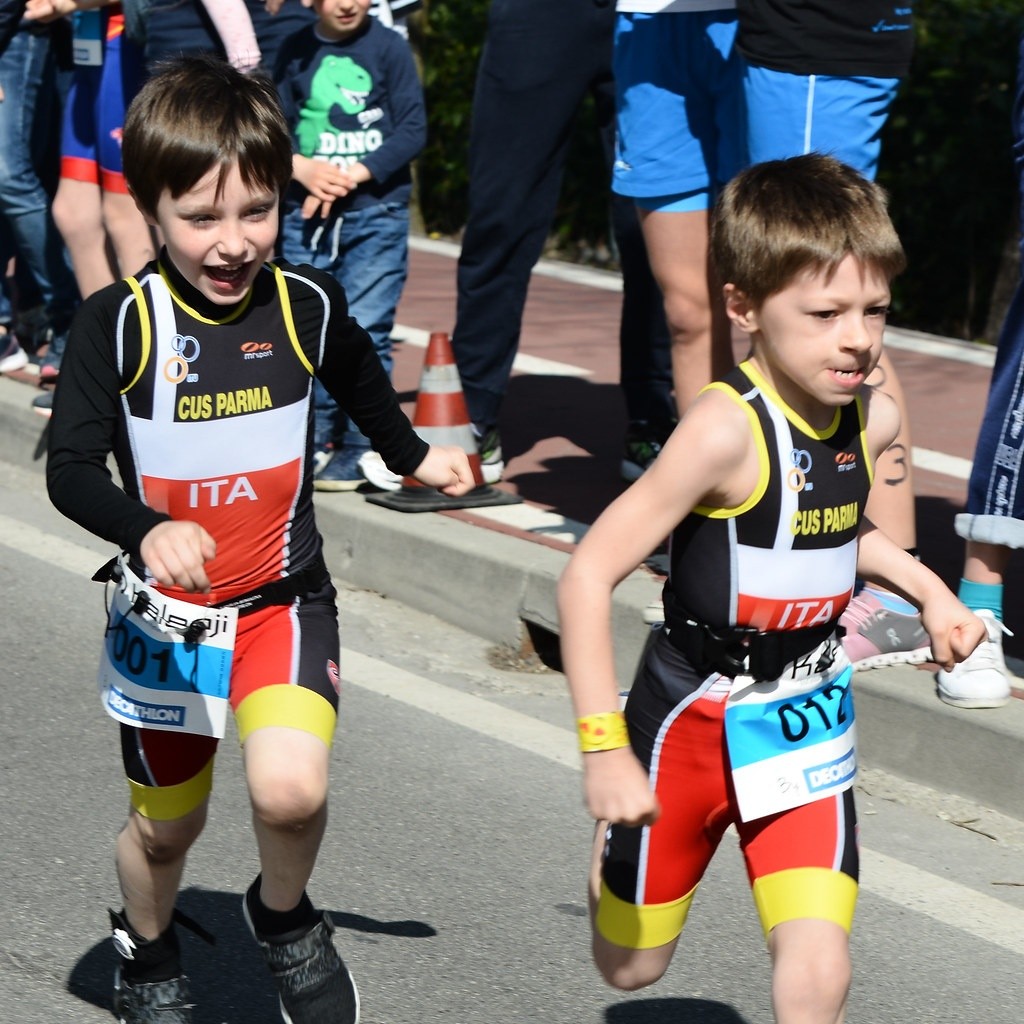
41;333;69;377
0;329;28;373
32;392;53;418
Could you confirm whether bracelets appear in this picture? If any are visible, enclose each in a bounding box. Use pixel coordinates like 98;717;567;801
575;711;631;753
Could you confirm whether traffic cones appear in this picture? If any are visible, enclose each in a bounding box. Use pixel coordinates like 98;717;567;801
366;331;524;514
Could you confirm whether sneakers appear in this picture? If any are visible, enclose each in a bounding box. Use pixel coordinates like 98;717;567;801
106;902;199;1024
241;871;359;1024
307;441;335;477
358;424;504;493
838;590;935;672
938;609;1012;709
312;445;375;491
620;432;664;483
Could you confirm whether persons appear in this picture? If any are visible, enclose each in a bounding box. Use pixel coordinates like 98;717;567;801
936;37;1024;709
715;0;937;672
611;0;752;576
272;0;428;492
358;0;680;493
554;150;990;1024
0;0;327;417
46;59;476;1024
